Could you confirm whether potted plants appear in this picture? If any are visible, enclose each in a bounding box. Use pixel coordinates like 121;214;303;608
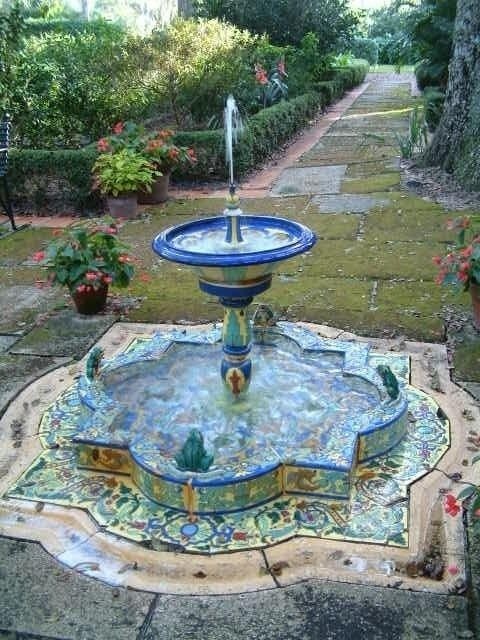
88;147;163;223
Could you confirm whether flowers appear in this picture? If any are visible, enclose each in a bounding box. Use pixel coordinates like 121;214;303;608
95;118;199;176
428;214;479;292
21;214;151;299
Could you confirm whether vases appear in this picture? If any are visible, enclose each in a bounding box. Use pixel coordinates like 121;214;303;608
136;163;173;206
69;281;110;317
465;281;479;330
